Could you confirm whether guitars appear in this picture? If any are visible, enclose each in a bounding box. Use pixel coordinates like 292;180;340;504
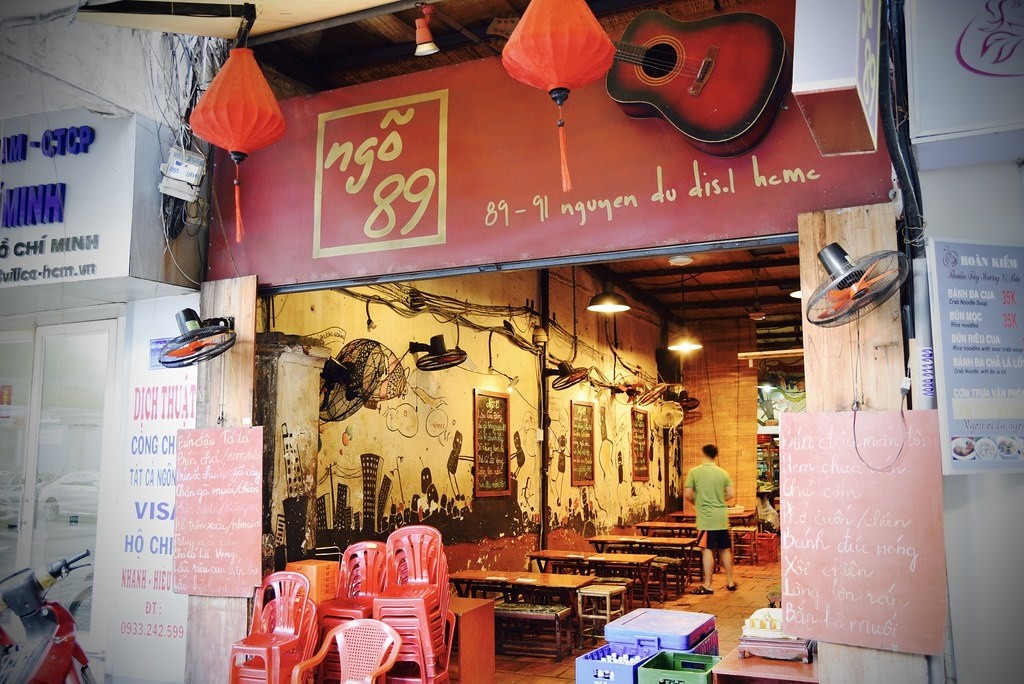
486;8;793;157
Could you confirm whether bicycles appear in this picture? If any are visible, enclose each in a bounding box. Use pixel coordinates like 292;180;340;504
69;569;92;653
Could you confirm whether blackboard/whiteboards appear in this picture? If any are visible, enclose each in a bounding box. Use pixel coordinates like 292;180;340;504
631;407;650;482
173;425;264;598
571;399;595;486
473;388;512;497
779;411;948;658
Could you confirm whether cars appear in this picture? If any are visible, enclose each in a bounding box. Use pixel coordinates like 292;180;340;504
40;470;99;522
0;471;58;522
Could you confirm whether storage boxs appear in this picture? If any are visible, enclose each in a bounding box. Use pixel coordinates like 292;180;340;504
637;650;723;684
575;642;662;684
741;533;779;551
741;546;779;563
284;559;340;604
685;628;719;656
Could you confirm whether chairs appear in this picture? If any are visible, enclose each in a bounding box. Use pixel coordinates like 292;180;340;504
228;526;456;684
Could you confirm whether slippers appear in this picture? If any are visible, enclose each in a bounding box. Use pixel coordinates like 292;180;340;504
726;582;736;590
689;585;713;594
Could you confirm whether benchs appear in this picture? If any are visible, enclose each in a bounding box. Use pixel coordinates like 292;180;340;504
653;557;684;598
591;576;633;613
493;602;573;664
606;544;704;582
652;528;698;538
551;561;668;603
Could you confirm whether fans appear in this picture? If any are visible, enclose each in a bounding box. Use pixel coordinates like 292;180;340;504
652;399;684;428
682;410;702;425
613;374;646;406
668;390;699;411
319;338;383;422
805;241;911;329
638;380;667;407
158;309;237;368
409;334;469;371
552;360;589;391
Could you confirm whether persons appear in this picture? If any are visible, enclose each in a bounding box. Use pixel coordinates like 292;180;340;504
684;443;737;595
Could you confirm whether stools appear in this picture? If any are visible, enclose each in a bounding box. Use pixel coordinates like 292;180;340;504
576;584;627;649
730;526;759;565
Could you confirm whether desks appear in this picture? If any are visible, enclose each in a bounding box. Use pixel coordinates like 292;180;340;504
447;509;757;652
712;646;819;684
448;598;495;684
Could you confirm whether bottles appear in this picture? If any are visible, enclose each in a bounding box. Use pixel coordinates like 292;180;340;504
594;653;641;679
659;677;685;684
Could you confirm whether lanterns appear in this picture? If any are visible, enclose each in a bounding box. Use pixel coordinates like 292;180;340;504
500;0;615;197
190;48;286;243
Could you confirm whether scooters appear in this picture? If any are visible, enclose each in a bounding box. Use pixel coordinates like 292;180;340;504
0;548;97;684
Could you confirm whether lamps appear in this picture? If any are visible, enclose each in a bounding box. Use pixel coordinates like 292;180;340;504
415;5;440;56
586;272;631;313
737;320;804;360
667;268;703;351
748;268;766;321
